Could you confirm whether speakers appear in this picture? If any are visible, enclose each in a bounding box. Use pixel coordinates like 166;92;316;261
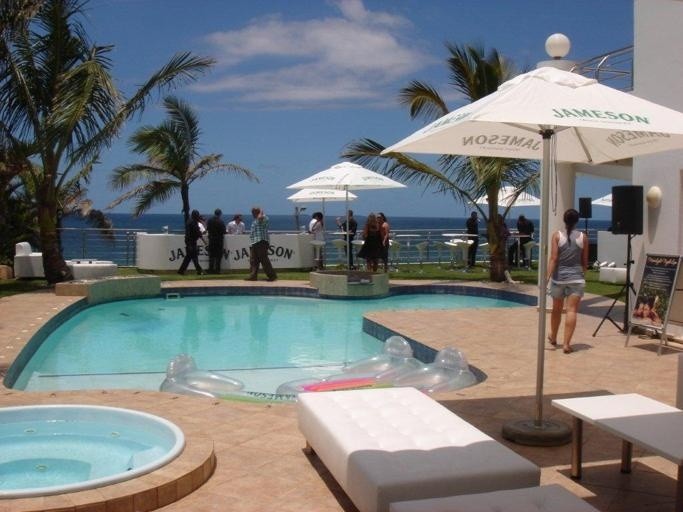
612;185;643;234
578;198;592;218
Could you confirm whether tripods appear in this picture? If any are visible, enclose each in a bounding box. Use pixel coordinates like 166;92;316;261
592;238;660;338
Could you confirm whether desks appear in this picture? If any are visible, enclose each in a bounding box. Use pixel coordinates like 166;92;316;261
551;393;683;512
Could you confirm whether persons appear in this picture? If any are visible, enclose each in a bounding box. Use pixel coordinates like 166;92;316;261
226;213;246;235
466;211;479;267
308;212;325;269
546;208;589;354
632;297;661;327
244;207;277;282
376;212;390;265
177;208;226;276
336;209;357;268
508;215;534;267
357;212;386;272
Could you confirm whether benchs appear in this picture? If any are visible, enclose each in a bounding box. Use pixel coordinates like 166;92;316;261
297;386;541;512
65;258;117;280
389;484;599;512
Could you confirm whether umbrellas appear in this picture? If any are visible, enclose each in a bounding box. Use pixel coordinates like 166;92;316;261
287;188;358;267
286;161;407;269
468;186;540;207
380;66;682;426
591;194;612;206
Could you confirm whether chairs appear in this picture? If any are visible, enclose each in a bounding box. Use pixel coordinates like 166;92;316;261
14;241;46;280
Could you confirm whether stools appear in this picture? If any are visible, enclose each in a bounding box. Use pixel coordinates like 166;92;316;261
310;234;536;273
